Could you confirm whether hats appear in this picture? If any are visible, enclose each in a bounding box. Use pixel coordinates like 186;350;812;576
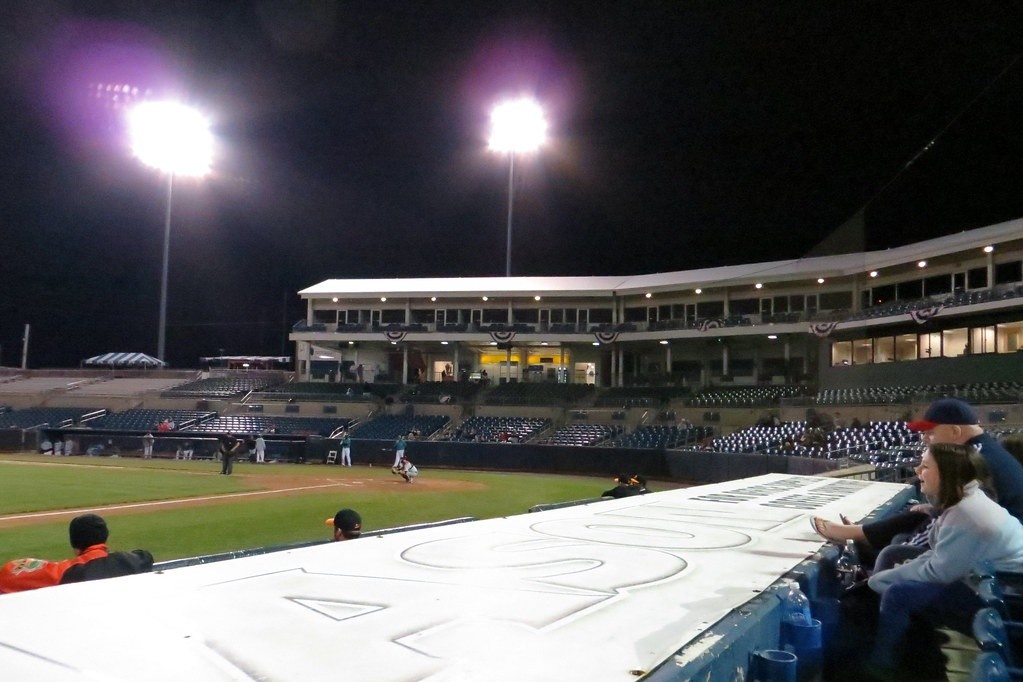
400;456;408;460
326;510;361;531
70;514;108;551
908;398;980;431
631;475;646;485
614;473;630;484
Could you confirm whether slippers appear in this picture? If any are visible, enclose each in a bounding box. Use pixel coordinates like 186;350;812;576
809;516;850;546
840;512;856;526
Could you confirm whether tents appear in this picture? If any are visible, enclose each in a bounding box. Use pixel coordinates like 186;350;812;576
201;354;290;370
81;350;168;378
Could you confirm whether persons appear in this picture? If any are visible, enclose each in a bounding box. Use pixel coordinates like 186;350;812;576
464;426;518;444
400;456;419;483
324;509;362;542
677;416;693;435
219;433;240;475
59;514;154;585
755;412;780;426
176;439;194;461
398;428;421;441
392;435;406;474
703;409;721;426
838;441;1023;682
345;364;372;400
609;410;627;425
433;427;454;442
780;430;828;456
142;430;154;460
40;436;74;457
809;397;1023;581
441;363;450;379
249;425;279;463
601;473;652;500
655;407;677;426
340;435;352;468
157;415;174;432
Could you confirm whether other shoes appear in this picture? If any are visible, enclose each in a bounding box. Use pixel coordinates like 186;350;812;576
406;477;413;482
218;471;226;474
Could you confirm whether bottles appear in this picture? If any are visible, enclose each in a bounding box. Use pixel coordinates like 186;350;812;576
834;550;853;588
843;539;859;565
787;582;814;627
